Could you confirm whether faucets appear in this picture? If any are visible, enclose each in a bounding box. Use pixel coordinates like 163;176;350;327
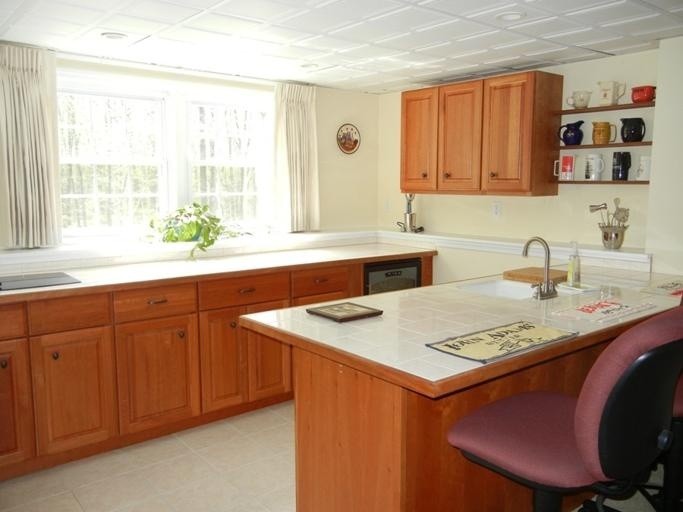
522;237;560;299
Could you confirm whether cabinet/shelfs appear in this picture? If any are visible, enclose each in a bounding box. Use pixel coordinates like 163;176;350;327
558;100;655;184
399;79;484;196
112;281;202;448
198;269;292;425
0;301;34;475
26;292;116;468
483;70;564;196
294;265;351;306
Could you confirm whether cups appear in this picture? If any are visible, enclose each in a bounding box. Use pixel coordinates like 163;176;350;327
584;153;604;181
553;154;576;183
613;151;631;181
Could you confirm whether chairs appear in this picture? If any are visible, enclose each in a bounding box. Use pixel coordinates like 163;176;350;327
446;306;683;512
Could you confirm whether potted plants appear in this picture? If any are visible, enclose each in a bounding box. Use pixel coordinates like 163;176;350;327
147;202;227;258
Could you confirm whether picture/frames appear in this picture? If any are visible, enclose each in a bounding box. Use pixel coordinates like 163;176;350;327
306;301;384;322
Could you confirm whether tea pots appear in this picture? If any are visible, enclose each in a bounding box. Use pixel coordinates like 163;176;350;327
596;79;625;105
630;86;655;102
616;118;646;142
591;120;616;144
565;90;592;109
558;120;582;146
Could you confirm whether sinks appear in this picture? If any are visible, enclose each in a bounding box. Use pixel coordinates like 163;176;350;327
457;277;567;302
